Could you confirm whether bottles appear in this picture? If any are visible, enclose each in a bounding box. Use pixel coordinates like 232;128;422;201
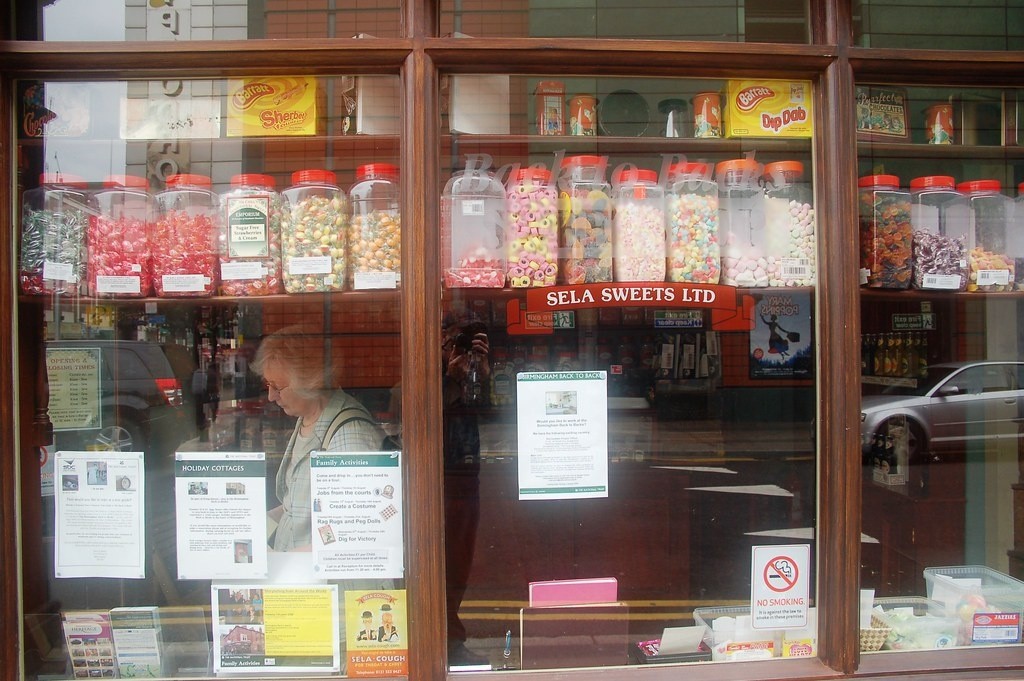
858;174;912;289
666;162;721;284
212;399;296;453
19;172;88;294
138;323;178;345
506;168;558;288
348;163;402;290
861;432;897;474
1014;182;1024;292
488;338;659;408
762;161;815;287
559;154;613;284
219;174;280;297
220;320;238;348
87;174;152;298
910;175;970;291
715;159;770;288
153;174;219;297
613;169;666;281
861;330;932;381
440;162;506;288
660;332;708;379
956;180;1016;292
280;170;348;294
375;412;400;445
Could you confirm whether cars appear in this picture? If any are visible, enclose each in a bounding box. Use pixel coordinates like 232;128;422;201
813;359;1024;462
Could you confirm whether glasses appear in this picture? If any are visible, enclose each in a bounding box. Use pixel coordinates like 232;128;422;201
261;377;289;391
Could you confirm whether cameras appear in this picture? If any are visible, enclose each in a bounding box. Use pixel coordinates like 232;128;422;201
452;333;483;354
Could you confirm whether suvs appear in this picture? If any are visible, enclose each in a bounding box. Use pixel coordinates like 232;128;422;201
45;341;201;468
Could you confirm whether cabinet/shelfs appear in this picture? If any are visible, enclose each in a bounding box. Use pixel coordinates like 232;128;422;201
0;0;1024;681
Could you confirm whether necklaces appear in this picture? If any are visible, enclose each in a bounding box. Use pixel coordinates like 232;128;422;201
301;416;320;428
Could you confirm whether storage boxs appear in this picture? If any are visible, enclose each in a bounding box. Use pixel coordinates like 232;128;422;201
721;79;816;140
227;77;325;136
439;32;510;135
341;34;401;135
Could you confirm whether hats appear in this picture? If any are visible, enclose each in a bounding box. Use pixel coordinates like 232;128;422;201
442;309;480;329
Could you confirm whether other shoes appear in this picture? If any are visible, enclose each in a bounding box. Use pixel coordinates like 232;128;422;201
448;644;491;672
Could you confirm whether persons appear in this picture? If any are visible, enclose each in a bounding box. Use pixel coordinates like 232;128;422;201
249;323;388;553
217;590;265;626
438;304;500;640
